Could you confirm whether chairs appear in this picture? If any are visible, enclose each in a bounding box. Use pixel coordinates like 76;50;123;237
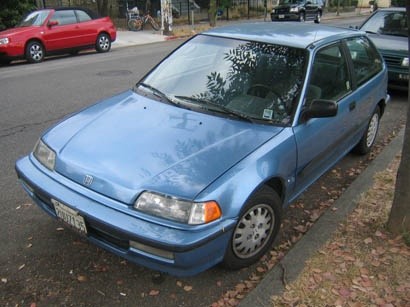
265;63;343;105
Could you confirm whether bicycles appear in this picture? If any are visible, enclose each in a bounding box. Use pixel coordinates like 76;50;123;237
127;10;160;32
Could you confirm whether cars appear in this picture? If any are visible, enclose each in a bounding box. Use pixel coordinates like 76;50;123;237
0;6;117;65
336;6;410;99
269;0;325;27
14;21;391;277
